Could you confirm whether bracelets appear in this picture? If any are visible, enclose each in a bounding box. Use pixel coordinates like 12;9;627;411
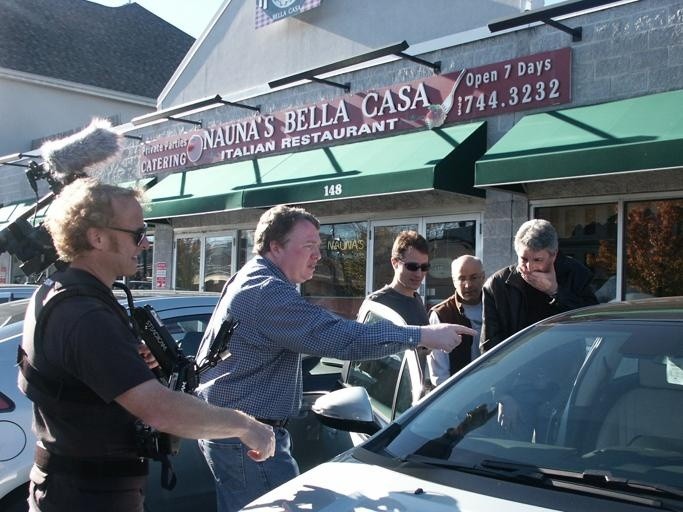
549;292;558;300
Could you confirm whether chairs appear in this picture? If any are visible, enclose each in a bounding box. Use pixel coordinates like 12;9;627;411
596;357;683;473
181;331;204;356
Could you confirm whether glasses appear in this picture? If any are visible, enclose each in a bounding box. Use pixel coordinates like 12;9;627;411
89;222;149;247
394;256;433;272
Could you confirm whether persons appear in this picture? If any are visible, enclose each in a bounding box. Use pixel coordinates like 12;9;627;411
425;255;495;435
17;179;276;511
353;229;435;414
480;219;598;445
193;207;479;511
584;251;608;295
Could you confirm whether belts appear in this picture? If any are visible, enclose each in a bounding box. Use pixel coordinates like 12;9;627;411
255;417;289;428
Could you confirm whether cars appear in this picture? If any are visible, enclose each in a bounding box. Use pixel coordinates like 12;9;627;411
0;289;355;512
235;296;683;511
